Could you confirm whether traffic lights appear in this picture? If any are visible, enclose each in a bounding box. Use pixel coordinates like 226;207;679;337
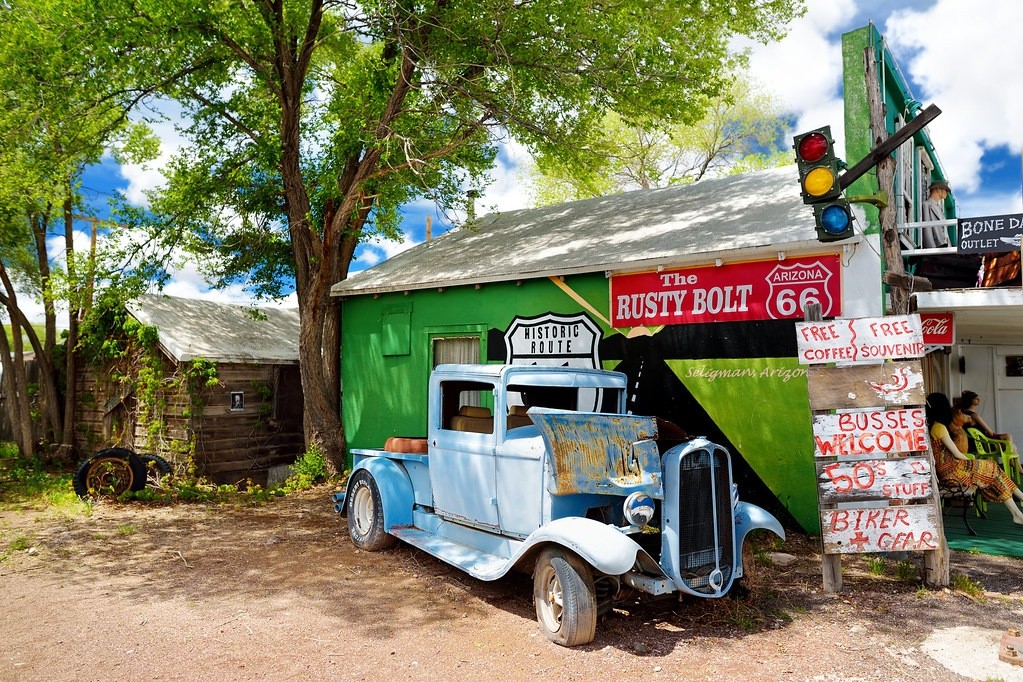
794;127;853;243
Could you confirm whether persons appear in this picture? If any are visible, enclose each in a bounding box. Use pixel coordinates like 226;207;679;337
961;390;1023;475
926;392;1023;524
922;181;951;248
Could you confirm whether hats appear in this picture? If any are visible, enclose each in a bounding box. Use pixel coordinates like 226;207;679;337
924;180;952;195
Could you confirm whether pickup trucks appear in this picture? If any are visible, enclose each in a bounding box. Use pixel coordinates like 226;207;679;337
333;364;787;647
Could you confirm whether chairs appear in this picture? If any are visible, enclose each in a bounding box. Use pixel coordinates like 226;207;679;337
453;405;494;434
941;427;1021;516
507;405;535;431
